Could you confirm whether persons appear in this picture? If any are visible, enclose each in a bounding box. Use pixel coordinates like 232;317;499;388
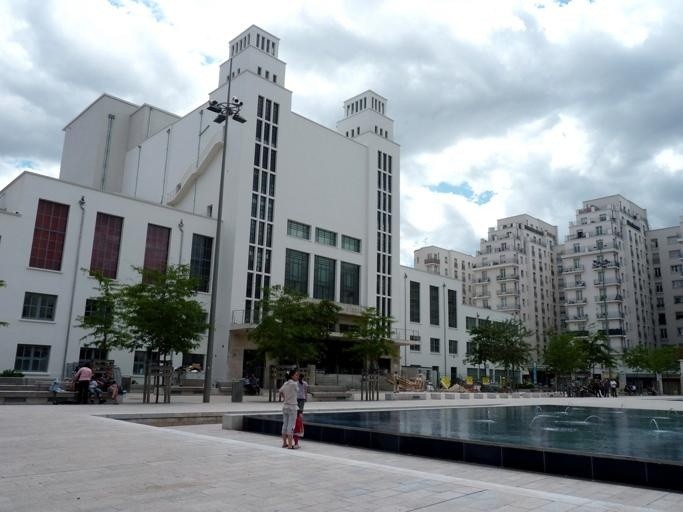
566;377;657;397
240;367;309;450
71;360;120;405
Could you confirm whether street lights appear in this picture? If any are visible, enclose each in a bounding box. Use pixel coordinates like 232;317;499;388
203;96;247;404
591;259;614;379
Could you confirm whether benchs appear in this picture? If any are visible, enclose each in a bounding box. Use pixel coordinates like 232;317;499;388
171;379;205;395
308;385;352;401
217;382;248;395
0;377;126;404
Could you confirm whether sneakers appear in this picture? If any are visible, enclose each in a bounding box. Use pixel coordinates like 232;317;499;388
281;443;302;450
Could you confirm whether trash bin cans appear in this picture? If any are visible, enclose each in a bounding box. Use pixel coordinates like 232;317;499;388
120;378;131;393
232;380;243;402
